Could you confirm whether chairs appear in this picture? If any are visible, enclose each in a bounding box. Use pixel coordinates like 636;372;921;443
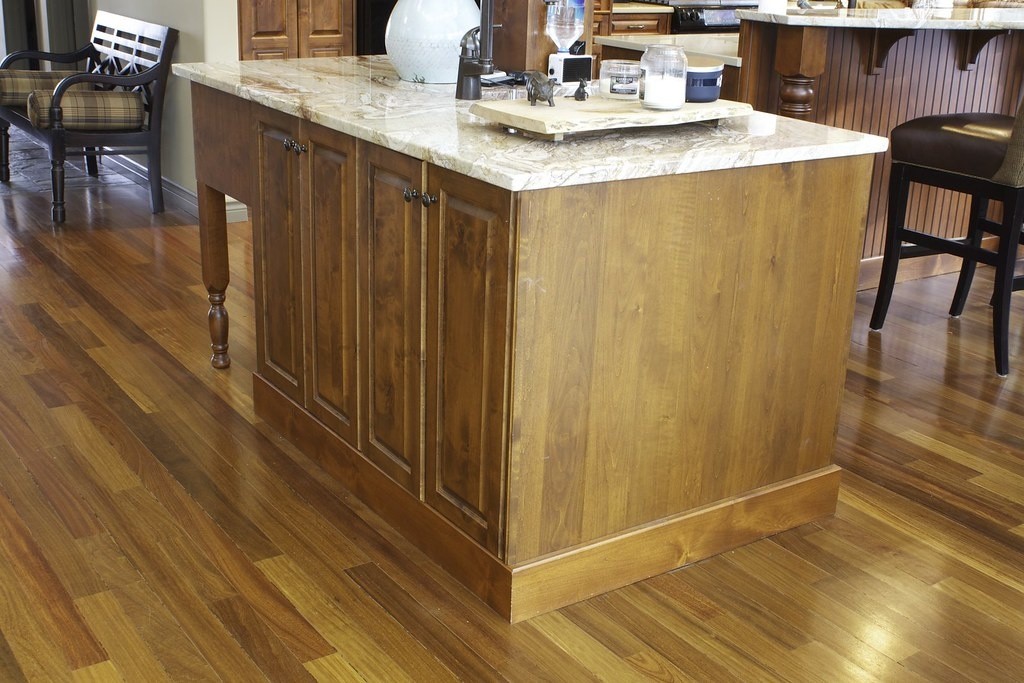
869;97;1024;375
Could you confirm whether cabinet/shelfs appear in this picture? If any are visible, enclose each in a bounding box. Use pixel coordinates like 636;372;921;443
592;0;674;80
171;55;890;627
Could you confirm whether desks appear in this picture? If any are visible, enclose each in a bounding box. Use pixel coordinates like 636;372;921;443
734;7;1024;121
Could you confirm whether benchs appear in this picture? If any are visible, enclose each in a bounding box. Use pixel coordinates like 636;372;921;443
0;9;179;226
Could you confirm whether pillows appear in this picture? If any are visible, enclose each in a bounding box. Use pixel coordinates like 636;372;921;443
27;89;146;130
0;69;95;107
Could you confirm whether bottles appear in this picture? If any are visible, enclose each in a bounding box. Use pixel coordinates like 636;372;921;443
544;0;586;54
637;43;689;110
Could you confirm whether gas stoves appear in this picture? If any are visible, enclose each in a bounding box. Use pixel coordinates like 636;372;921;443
643;0;759;35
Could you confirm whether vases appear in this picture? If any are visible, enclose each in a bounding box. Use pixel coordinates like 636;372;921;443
385;0;480;84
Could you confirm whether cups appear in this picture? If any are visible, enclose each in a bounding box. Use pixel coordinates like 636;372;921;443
680;61;725;103
597;59;641;99
